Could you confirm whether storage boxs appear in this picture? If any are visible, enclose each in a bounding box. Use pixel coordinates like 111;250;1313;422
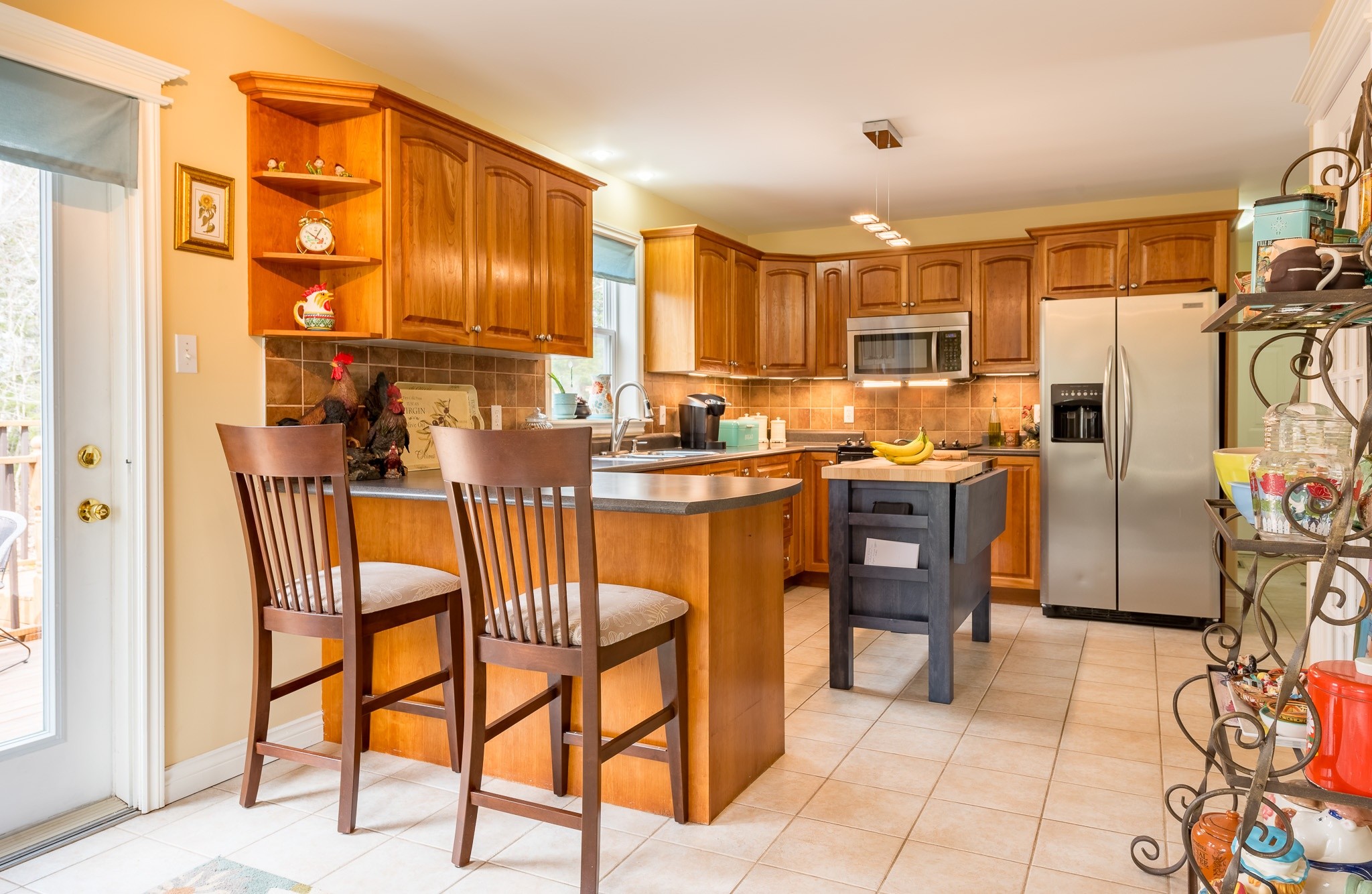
1304;662;1372;797
720;420;759;446
1251;193;1338;310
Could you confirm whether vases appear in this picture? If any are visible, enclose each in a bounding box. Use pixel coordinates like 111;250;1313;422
588;374;613;419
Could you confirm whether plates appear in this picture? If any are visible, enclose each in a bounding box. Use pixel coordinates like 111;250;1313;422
1227;680;1306;749
1230;835;1310;884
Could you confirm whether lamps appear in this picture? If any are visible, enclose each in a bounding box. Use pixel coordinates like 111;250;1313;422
849;120;911;247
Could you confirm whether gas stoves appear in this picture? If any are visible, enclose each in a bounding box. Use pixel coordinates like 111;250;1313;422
838;437;981;464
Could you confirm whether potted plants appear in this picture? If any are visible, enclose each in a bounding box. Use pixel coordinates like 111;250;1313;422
549;373;591;420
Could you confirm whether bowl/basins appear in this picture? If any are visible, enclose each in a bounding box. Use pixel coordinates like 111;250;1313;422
1266;700;1307;725
1213;446;1264;505
1258;706;1307;739
1227;481;1256;524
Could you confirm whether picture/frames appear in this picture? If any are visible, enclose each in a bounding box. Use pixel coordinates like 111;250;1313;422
174;163;235;260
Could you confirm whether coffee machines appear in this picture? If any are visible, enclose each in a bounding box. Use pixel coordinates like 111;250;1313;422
678;392;732;450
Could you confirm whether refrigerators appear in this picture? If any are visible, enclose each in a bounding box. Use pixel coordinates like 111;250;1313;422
1039;291;1221;632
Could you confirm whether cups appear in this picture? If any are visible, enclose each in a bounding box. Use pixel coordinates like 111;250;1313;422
1262;238;1342;292
738;413;754;420
1003;426;1020;447
752;412;769;443
770;417;786;443
1316;242;1366;290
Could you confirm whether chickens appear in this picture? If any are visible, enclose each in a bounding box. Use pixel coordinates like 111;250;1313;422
272;353;361;462
293;281;335;332
1022;405;1040;442
383;440;400;476
362;371;412;477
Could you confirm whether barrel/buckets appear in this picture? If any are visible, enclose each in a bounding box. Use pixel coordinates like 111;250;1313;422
1304;659;1372;798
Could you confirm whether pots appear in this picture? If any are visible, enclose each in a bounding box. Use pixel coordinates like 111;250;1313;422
1262;779;1372;894
1190;810;1243;883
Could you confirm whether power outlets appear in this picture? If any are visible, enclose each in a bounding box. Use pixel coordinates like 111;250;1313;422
659;406;666;425
843;406;854;423
491;405;502;430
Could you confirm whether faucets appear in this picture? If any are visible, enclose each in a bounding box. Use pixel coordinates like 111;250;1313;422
608;381;655;453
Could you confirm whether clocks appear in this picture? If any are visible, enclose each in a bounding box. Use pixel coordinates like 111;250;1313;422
296;209;336;255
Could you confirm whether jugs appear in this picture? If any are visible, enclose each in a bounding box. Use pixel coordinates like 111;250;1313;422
292;282;335;331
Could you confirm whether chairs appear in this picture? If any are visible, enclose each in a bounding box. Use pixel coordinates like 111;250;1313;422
430;424;688;894
0;511;30;672
215;423;465;834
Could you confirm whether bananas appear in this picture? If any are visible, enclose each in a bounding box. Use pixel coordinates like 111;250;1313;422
870;427;934;465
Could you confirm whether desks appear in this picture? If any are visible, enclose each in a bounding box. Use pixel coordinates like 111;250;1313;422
821;456;1008;705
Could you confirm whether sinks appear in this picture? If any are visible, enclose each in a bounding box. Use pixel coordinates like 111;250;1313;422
615;450;719;460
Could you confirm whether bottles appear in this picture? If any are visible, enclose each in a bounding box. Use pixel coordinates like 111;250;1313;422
586;371;614;419
1248;402;1353;543
988;390;1001;447
519;407;554;430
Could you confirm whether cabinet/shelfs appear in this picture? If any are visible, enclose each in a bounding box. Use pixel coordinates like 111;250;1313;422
850;244;1039;375
247;91;593;358
1131;69;1372;893
645;235;849;378
991;455;1041;591
663;453;837;580
1037;218;1228;302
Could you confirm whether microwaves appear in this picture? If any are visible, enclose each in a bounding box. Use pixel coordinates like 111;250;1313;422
846;311;972;382
